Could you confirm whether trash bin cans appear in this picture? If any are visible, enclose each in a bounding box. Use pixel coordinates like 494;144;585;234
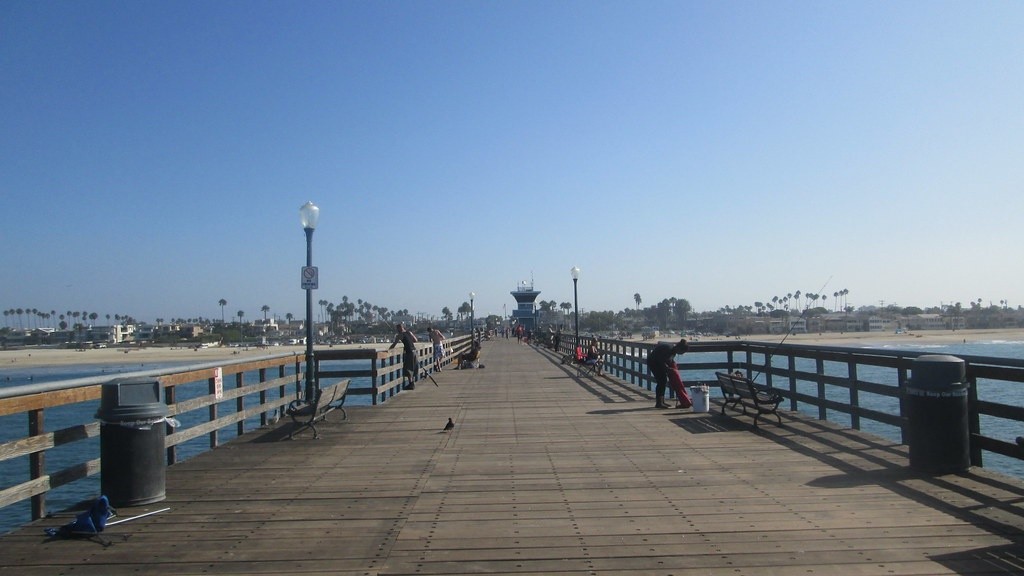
94;377;167;508
901;355;971;472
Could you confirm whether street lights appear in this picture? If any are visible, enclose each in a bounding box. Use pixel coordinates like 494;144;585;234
570;266;581;358
469;290;476;355
533;300;538;332
297;200;319;406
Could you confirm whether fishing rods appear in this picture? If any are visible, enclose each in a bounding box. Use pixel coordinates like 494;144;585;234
585;314;638;376
731;274;836;411
377;312;439;387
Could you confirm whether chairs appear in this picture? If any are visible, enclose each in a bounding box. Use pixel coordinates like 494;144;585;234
576;344;597;375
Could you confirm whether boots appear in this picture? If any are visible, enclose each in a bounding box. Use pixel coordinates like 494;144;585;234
655;395;671;408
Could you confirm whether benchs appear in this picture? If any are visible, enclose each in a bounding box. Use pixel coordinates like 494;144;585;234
460;355;480;370
287;380;350;439
714;372;783;428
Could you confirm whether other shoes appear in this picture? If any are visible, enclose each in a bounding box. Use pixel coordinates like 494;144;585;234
454;366;461;370
403;382;415;390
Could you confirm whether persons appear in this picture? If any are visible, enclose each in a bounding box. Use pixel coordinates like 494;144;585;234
649;339;688;408
390;324;418;390
454;342;481;369
555;330;562;353
478;328;510;343
427;327;445;372
586;338;605;376
512;324;544;344
546;325;554;349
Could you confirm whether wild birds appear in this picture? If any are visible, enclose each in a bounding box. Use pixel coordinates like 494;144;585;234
443;417;455;432
